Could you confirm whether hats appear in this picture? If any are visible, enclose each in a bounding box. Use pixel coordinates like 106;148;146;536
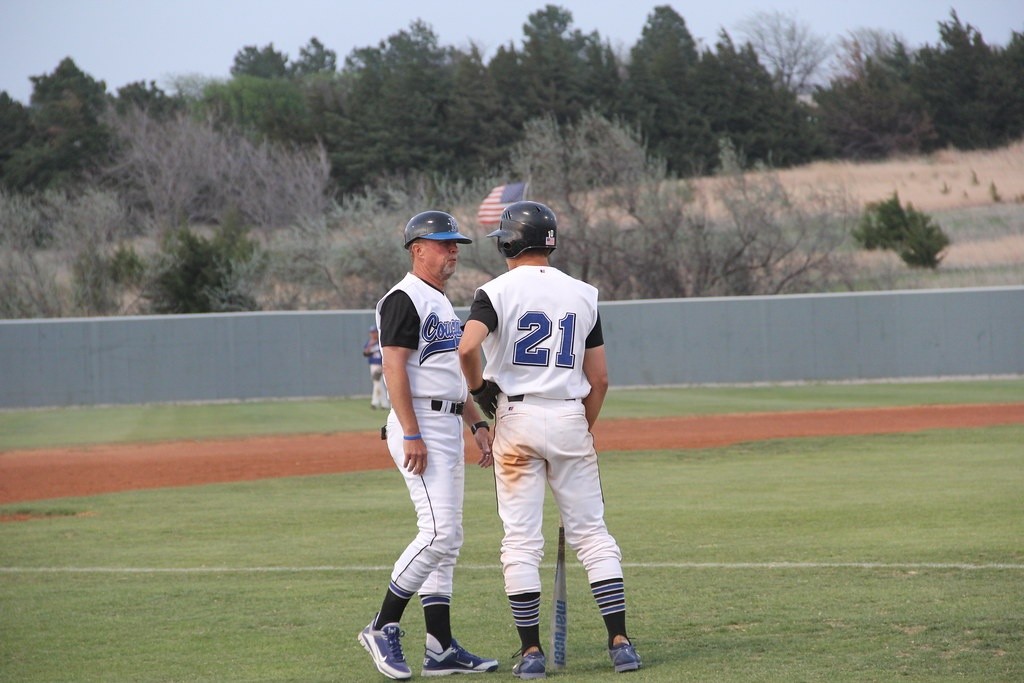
369;325;377;332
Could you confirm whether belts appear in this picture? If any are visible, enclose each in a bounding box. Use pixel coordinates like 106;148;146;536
508;394;574;402
432;400;464;414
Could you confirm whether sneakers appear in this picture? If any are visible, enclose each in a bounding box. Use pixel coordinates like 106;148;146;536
608;637;642;672
358;612;412;680
421;633;498;677
512;648;547;680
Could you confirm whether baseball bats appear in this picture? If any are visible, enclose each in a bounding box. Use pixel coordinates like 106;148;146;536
548;511;568;672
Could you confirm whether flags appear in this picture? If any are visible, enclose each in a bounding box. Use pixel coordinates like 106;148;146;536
478;183;525;223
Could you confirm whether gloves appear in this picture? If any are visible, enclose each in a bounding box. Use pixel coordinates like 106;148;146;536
470;379;501;420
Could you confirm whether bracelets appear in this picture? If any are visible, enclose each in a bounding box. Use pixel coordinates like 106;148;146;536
404;433;421;440
471;421;489;435
468;378;487;396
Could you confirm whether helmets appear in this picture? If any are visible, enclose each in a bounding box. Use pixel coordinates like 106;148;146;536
486;201;558;258
404;211;472;250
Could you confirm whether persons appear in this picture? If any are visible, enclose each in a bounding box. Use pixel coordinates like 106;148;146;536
357;210;499;680
460;200;643;679
362;325;391;410
